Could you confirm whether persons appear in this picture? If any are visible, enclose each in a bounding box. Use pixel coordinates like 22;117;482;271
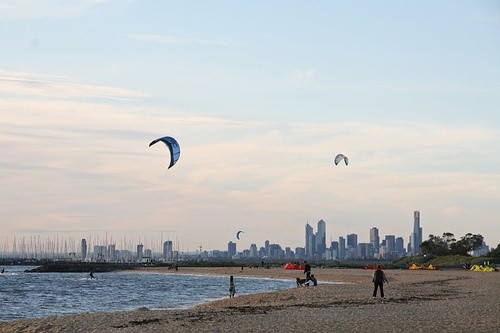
89;272;95;279
228;276;235;298
303;261;317;287
372;264;388;299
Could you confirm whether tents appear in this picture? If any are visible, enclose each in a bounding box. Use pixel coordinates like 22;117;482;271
427;264;437;270
470;265;495;271
285;262;304;270
409;264;422;270
